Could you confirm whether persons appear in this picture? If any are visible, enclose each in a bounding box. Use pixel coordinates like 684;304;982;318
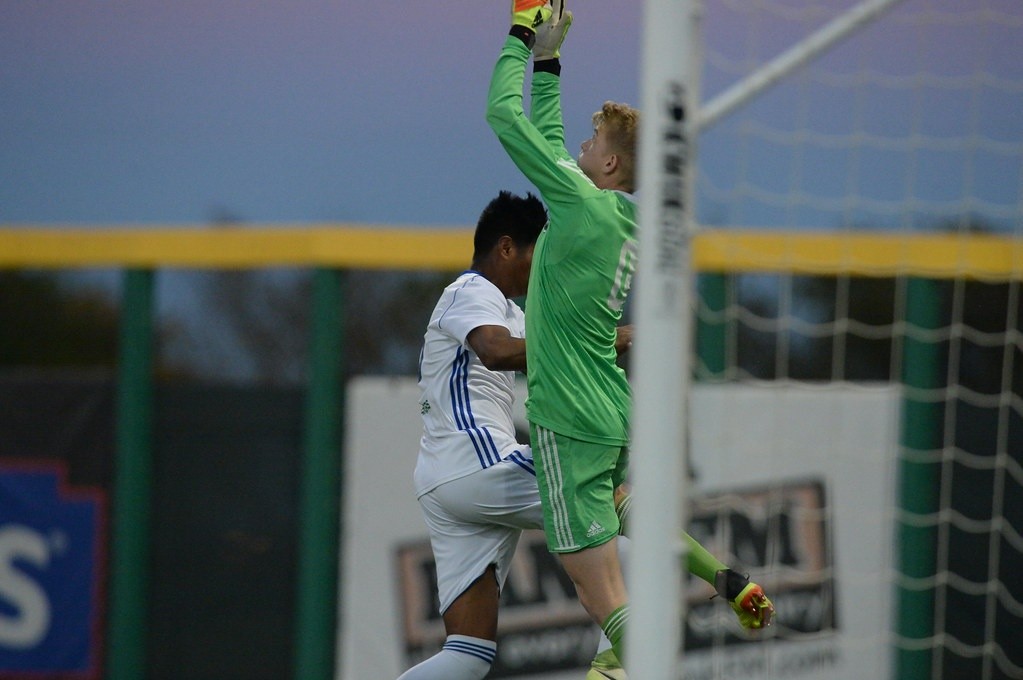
486;0;776;680
397;190;635;680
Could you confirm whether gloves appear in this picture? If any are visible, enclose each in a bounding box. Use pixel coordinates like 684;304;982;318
511;0;553;36
532;0;573;62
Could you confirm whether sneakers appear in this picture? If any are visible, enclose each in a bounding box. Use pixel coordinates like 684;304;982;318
709;569;776;631
